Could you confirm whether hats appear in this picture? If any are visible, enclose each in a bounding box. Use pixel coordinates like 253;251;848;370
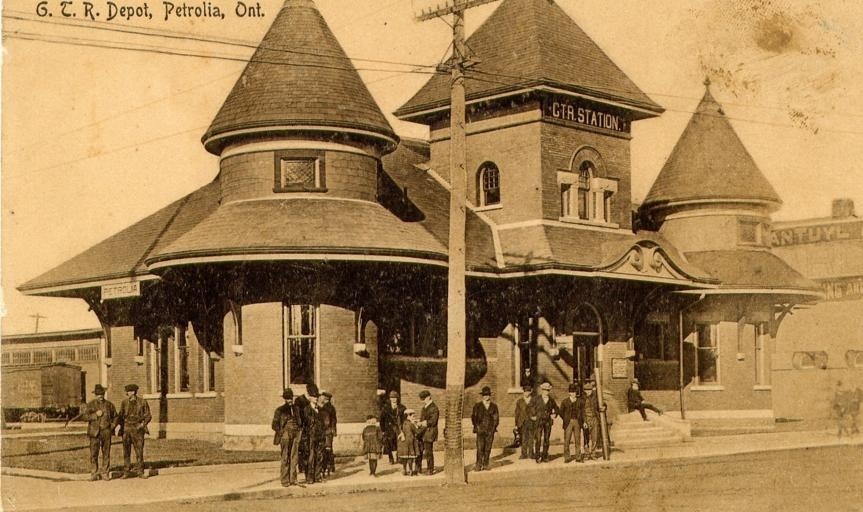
124;384;139;391
478;386;491;395
631;379;640;384
283;384;332;397
91;384;108;394
569;383;592;392
523;383;552;391
367;413;378;418
388;390;430;415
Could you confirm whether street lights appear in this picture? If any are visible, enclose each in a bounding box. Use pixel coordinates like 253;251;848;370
679;292;707;443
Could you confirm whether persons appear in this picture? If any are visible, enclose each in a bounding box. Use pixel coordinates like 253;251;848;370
82;384;118;481
628;378;662;420
118;384;152;479
272;382;607;487
